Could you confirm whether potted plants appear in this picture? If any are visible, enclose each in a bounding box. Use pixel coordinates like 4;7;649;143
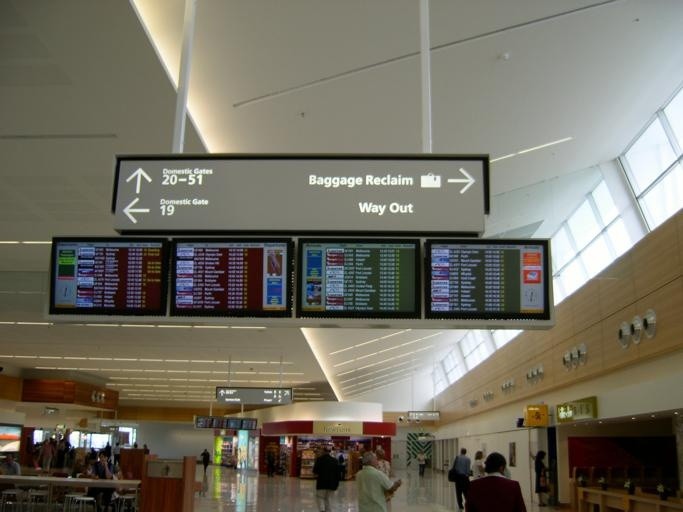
577;473;668;500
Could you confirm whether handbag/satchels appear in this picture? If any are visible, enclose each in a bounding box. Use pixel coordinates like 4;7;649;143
446;468;457;481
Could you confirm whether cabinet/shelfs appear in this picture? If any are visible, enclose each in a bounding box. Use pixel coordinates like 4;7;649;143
299;448;315;479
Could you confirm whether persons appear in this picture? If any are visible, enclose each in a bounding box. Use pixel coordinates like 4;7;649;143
264;446;274;477
471;450;483;479
533;449;549;506
28;436;149;470
311;447;340;512
463;451;527;512
354;450;401;512
84;449;116;511
450;447;472;509
373;448;392;512
200;448;209;472
328;446;347;481
0;452;20;474
416;450;427;477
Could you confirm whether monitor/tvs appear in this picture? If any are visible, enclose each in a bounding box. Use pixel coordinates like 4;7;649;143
422;237;555;329
197;417;257;430
298;237;422;329
172;237;297;325
45;236;171;323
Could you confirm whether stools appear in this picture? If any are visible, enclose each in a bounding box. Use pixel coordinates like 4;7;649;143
0;474;142;512
